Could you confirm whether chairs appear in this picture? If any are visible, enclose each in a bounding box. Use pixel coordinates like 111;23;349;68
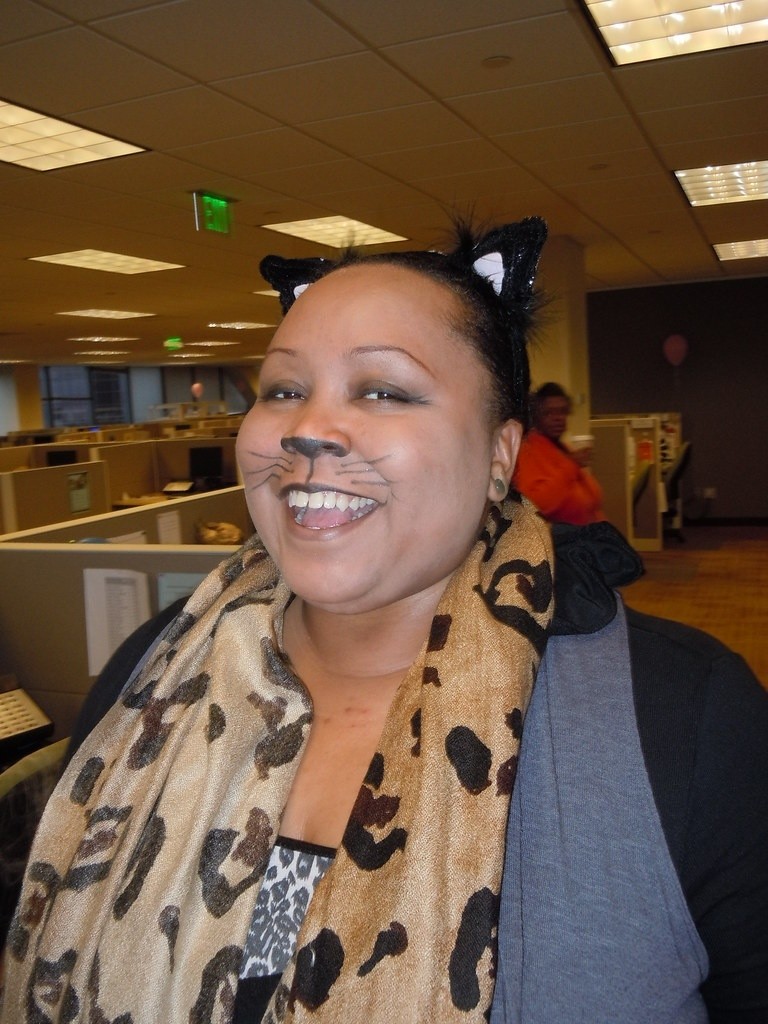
632;457;653;506
663;442;691;531
1;736;73;962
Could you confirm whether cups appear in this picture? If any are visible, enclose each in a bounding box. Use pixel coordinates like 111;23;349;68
571;435;595;466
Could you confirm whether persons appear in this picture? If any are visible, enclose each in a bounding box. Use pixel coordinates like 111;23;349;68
2;218;766;1022
510;383;608;526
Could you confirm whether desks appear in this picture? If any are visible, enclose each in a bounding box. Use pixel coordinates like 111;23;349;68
1;413;681;745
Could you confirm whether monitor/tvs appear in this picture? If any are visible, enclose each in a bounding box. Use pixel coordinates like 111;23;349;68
33;435;54;444
46;450;76;466
189;446;223;490
